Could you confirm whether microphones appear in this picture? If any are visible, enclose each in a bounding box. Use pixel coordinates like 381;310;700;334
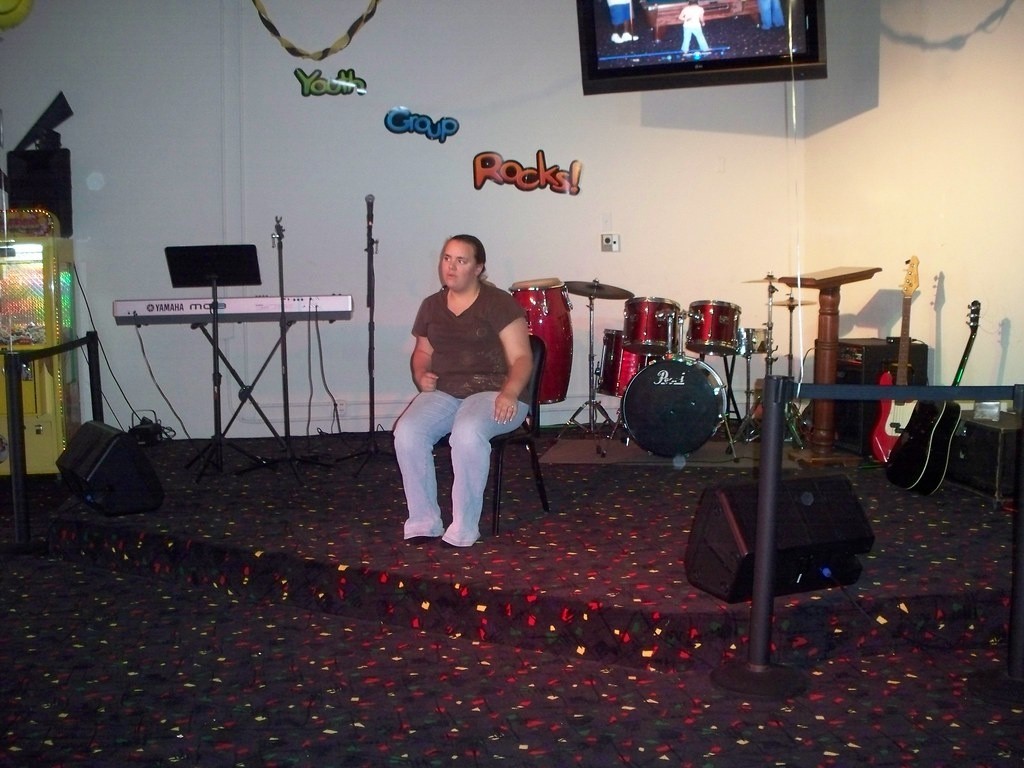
365;193;375;225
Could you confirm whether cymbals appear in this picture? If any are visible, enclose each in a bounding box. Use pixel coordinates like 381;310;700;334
766;300;817;306
563;278;635;302
740;278;779;283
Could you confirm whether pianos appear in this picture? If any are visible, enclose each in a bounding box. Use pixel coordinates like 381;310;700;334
111;292;355;486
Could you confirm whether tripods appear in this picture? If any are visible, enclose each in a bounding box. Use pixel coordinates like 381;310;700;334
551;295;627;456
724;281;811;456
164;215;394;485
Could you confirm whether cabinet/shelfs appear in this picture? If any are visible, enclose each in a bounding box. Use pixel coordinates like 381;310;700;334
946;410;1018;504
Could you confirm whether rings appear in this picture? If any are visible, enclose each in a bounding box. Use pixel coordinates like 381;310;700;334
508;405;514;409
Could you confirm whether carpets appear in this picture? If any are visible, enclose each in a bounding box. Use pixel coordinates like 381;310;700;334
539;439;800;469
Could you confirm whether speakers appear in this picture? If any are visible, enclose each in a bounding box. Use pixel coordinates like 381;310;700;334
56;421;164;517
7;148;72;239
685;472;875;604
945;410;1018;504
816;338;929;456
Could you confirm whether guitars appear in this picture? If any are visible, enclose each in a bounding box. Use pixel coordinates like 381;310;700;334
870;255;922;465
884;299;983;496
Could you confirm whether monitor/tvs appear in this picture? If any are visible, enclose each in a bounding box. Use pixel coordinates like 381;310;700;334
576;0;827;97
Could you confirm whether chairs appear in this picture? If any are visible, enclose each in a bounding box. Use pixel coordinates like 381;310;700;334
437;335;550;536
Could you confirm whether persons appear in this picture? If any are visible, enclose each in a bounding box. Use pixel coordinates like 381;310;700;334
394;235;532;547
606;0;788;57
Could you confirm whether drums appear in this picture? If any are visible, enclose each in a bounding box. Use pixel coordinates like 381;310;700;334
597;328;654;399
736;327;768;354
685;299;742;357
508;277;574;406
620;356;728;458
623;297;682;357
